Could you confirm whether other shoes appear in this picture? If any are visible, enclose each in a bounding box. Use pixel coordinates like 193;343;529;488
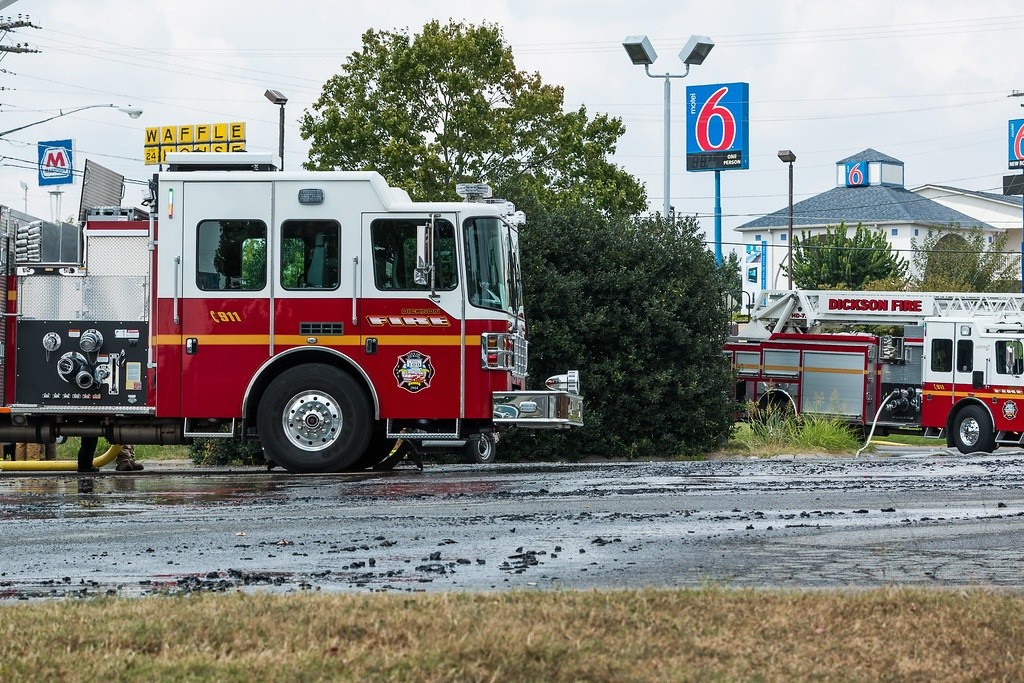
78;465;100;472
116;460;134;471
130;462;144;470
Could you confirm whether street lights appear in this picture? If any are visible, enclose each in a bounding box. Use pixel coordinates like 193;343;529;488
620;33;717;223
777;149;798;289
265;89;289;171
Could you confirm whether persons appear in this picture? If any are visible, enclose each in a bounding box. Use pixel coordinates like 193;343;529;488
115;445;144;471
74;435;100;472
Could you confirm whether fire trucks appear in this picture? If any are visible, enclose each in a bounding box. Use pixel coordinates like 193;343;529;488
712;284;1024;457
1;151;587;474
